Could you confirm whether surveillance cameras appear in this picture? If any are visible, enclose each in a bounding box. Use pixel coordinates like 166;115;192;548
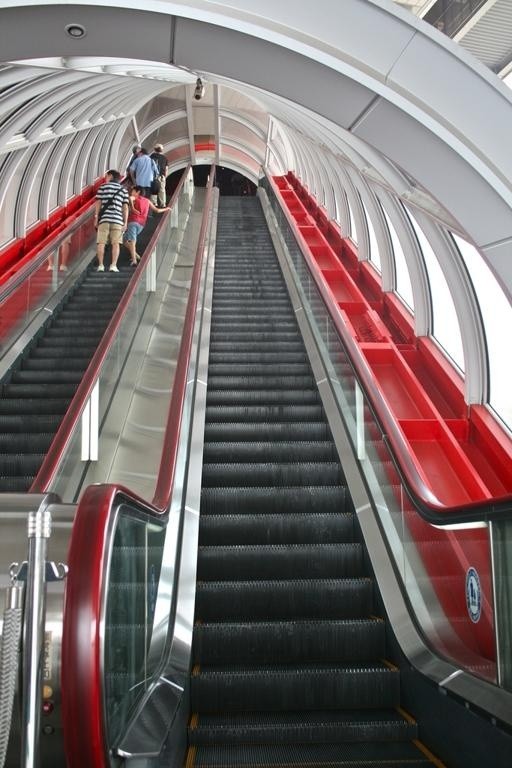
194;85;205;101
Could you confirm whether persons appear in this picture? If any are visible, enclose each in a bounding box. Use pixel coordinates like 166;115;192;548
130;148;160;220
150;143;169;209
127;146;142;185
123;184;174;267
95;169;130;272
47;235;72;273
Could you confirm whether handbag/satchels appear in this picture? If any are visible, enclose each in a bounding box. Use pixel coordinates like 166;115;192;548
150;179;161;195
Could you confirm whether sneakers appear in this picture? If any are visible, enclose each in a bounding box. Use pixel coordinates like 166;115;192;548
97;264;105;273
108;264;119;273
